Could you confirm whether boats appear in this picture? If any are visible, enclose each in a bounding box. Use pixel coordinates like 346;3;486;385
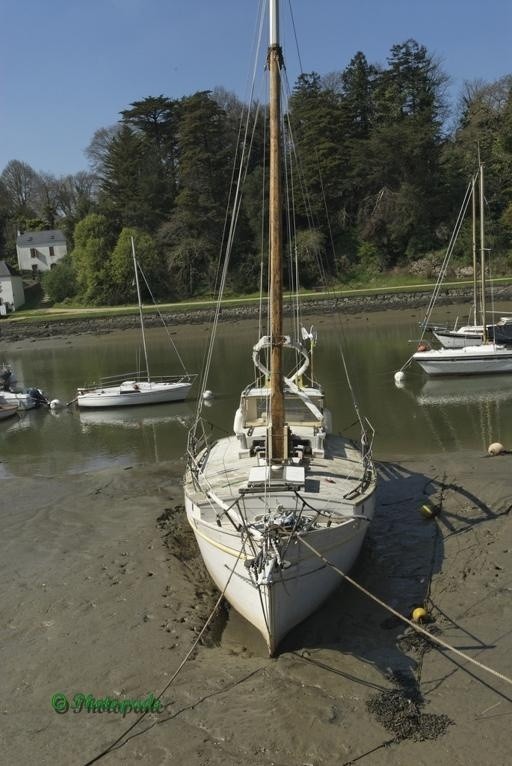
77;236;198;406
0;364;43;419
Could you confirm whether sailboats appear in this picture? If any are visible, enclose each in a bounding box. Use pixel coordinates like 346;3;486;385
413;163;512;375
183;0;377;657
